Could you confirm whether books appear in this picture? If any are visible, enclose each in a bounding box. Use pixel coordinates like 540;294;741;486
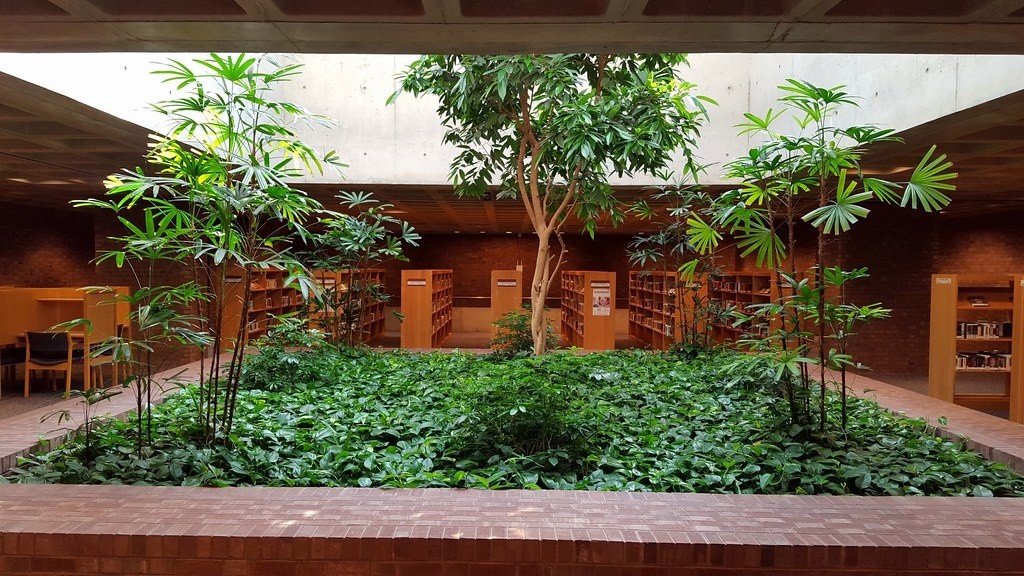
432;279;451;332
1008;296;1013;305
712;314;769;337
341;279;384;331
630;280;675;337
713;280;770;293
956;320;1012;339
711;298;770;316
249;279;308;331
967;296;989;307
561;280;584;347
956;350;1011;370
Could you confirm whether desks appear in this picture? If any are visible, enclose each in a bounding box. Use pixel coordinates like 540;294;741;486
0;284;133;392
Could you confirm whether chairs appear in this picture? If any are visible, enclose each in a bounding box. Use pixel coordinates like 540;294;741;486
0;323;125;398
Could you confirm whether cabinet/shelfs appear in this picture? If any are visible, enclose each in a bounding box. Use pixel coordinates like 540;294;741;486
401;269;454;347
705;270;796;358
491;268;523;350
223;260;308;351
308;268;386;349
928;273;1024;425
624;272;708;352
559;270;617;352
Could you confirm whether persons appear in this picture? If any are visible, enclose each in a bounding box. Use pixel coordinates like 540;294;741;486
593;297;610;307
